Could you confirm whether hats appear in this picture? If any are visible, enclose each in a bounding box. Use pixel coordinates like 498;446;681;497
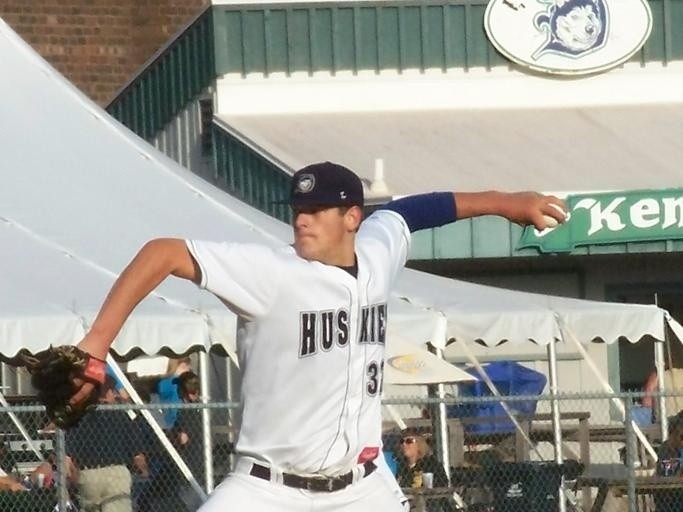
269;161;364;205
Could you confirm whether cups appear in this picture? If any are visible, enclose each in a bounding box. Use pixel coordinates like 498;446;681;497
661;459;673;478
422;472;433;490
35;473;44;489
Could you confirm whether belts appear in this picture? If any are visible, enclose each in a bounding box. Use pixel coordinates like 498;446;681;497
80;462;122;470
250;460;377;492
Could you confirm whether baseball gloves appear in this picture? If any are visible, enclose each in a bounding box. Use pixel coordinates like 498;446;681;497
26;344;108;428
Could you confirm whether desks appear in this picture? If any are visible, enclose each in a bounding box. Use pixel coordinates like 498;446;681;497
614;476;682;512
401;486;467;512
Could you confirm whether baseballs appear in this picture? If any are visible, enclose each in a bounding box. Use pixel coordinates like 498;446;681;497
544;203;563;228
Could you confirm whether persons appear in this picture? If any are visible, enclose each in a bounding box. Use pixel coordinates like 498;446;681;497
0;353;215;493
26;159;569;512
391;425;448;488
642;359;682;432
651;409;682;512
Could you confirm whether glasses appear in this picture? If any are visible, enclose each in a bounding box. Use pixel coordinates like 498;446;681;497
399;438;415;444
